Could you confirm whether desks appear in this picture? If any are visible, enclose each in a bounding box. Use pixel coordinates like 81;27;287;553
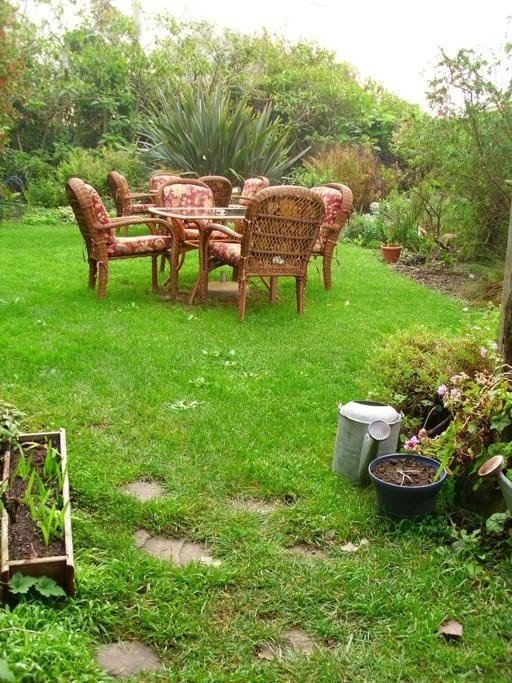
147;206;249;303
0;200;28;228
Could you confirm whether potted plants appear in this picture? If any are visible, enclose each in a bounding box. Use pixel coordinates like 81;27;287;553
361;324;493;436
1;429;75;602
377;193;429;264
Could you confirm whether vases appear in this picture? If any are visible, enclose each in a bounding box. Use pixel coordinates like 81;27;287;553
457;471;506;519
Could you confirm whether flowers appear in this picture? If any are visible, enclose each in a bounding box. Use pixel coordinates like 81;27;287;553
402;340;512;485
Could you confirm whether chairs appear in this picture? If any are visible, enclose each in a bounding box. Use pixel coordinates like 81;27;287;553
67;178;179;306
247;181;352;306
157;179;237;282
106;171;159;237
199;184;326;323
150;170;197;203
225;177;270;233
200;177;233;227
